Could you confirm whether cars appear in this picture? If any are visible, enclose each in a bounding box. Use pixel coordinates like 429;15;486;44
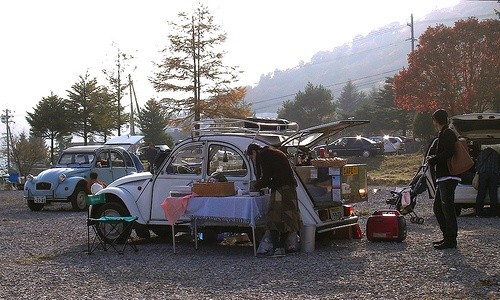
24;134;144;212
399;136;411;142
88;117;371;250
423;112;500;215
191;147;234;160
313;137;384;158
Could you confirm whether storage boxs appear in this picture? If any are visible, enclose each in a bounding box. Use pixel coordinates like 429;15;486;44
191;182;235;197
295;166;317;178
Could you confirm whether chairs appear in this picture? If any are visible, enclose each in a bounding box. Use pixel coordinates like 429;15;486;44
87;194;139;255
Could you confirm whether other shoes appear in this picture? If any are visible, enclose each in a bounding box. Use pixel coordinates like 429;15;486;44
266;248;286;257
432;239;444;244
434;241;457;249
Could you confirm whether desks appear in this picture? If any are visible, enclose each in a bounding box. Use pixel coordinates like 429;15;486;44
161;194;274;256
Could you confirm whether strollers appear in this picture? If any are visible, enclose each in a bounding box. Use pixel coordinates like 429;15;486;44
387;165;429;225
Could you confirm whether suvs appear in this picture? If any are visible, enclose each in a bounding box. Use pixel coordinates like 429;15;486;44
371;136;405;155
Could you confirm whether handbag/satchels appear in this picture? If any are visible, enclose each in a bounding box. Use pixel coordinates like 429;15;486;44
472;172;480;190
442;129;474;175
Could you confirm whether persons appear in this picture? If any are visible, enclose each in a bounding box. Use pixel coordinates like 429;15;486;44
246;144;301;258
86;172;105;193
425;109;463;249
468;148;500;217
146;144;156;174
